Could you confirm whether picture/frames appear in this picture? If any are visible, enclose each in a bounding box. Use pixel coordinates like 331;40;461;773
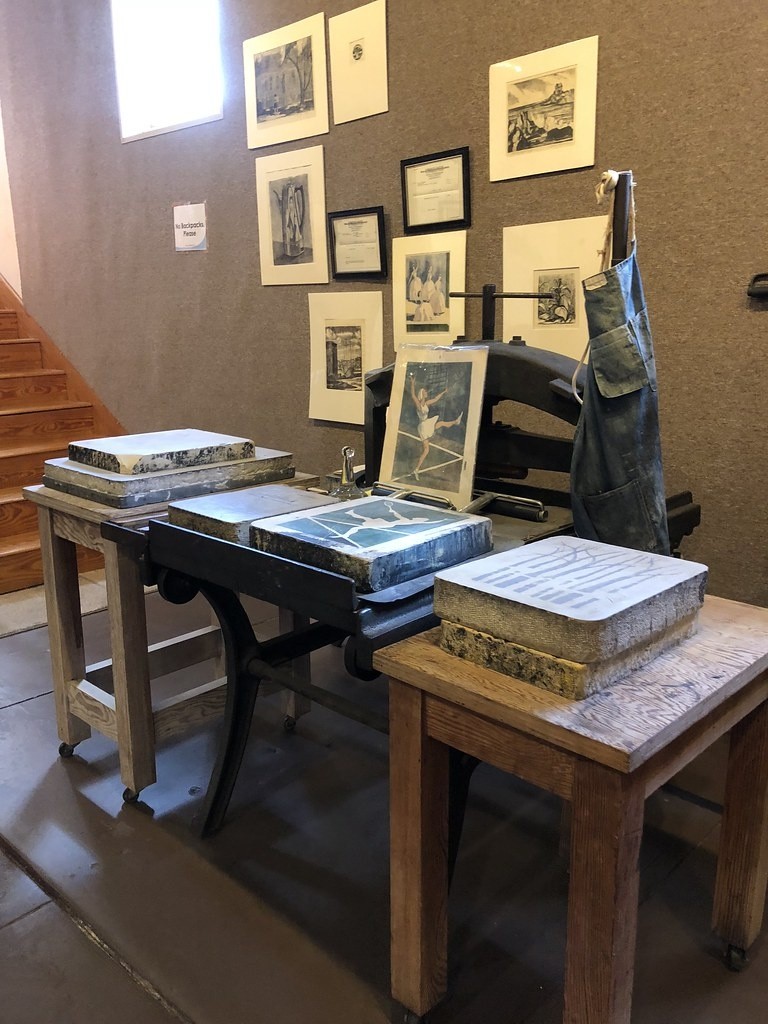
400;146;471;234
327;205;388;280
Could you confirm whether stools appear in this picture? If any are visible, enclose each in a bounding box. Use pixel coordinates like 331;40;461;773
372;594;768;1024
22;472;318;803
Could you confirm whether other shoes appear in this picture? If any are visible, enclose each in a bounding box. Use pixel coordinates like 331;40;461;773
413;469;421;481
